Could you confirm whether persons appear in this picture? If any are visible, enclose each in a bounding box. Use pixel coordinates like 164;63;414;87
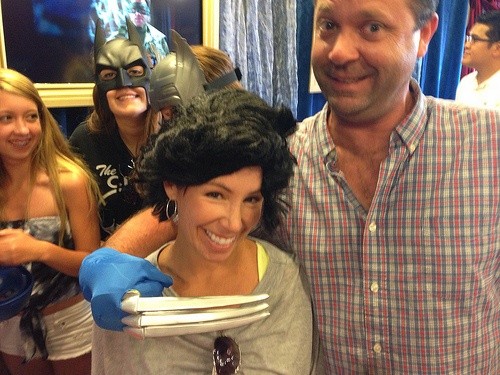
66;15;314;375
105;0;169;68
78;0;500;375
0;67;107;375
455;10;500;110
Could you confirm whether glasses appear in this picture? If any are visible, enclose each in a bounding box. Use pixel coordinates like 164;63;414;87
465;35;500;45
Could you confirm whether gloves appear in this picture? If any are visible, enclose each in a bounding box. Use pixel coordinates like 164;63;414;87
78;247;173;331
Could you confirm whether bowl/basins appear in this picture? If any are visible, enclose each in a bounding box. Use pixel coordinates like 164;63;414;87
0;265;33;321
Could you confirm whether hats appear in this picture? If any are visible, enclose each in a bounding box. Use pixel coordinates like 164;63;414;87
93;15;152;112
149;30;242;115
